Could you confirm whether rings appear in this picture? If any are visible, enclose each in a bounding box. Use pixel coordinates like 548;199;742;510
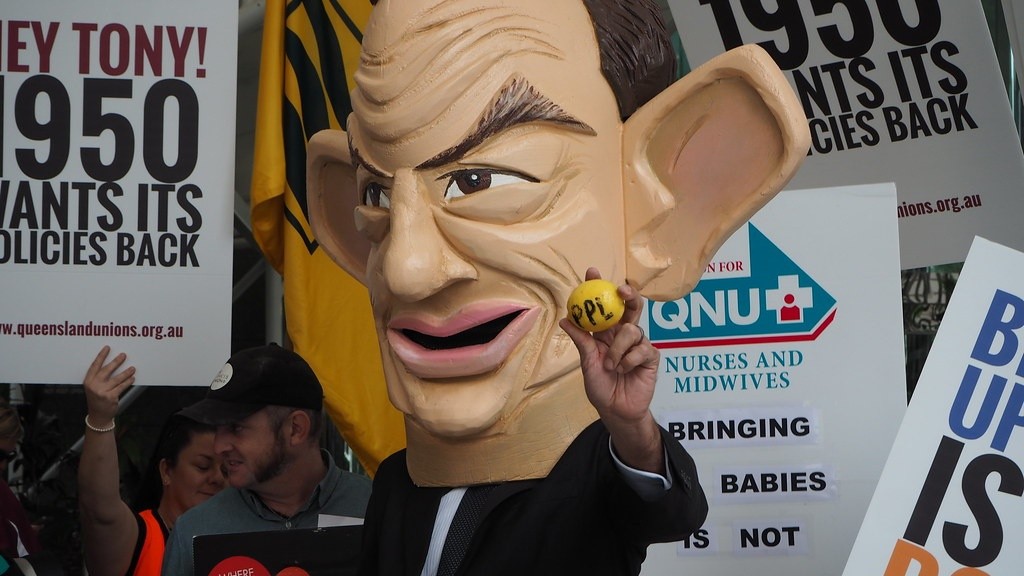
636;325;644;346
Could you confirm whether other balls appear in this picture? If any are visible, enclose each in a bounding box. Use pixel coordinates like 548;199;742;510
564;276;627;334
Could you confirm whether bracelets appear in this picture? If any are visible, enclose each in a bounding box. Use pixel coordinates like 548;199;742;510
85;415;115;431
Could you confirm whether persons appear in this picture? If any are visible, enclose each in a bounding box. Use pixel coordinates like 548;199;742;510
307;0;812;576
0;343;374;576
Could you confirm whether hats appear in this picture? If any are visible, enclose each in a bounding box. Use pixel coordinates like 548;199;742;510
176;341;324;428
